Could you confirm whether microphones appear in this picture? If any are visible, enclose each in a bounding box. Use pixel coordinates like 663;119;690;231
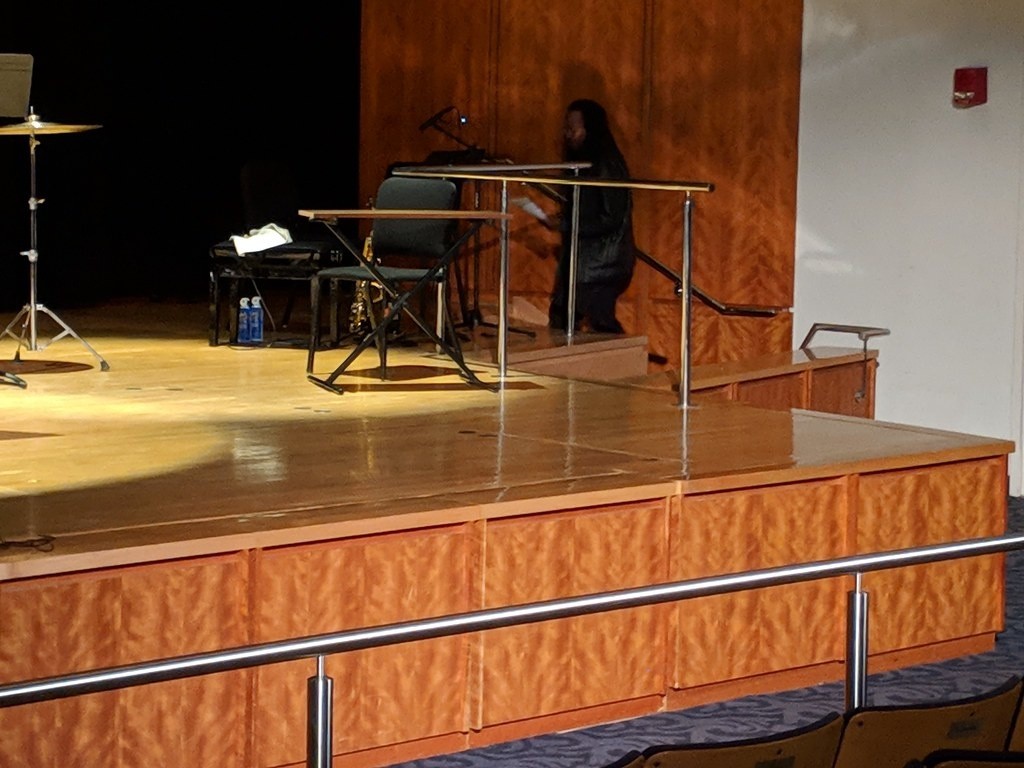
418;106;454;131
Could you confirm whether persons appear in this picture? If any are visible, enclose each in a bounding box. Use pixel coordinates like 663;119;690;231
537;98;633;333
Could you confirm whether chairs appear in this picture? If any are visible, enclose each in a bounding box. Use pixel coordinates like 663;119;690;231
306;177;467;380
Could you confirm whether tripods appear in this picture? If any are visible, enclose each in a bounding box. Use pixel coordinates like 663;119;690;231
432;125;536;339
0;134;110;370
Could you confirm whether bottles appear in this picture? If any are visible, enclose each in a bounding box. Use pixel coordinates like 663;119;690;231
237;296;263;342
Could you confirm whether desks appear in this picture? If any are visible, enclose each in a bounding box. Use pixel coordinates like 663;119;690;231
298;209;511;396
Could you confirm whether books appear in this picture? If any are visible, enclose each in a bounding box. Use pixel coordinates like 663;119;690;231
512;196;547;222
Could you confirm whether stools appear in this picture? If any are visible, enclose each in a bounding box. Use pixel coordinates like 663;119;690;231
207;238;341;347
